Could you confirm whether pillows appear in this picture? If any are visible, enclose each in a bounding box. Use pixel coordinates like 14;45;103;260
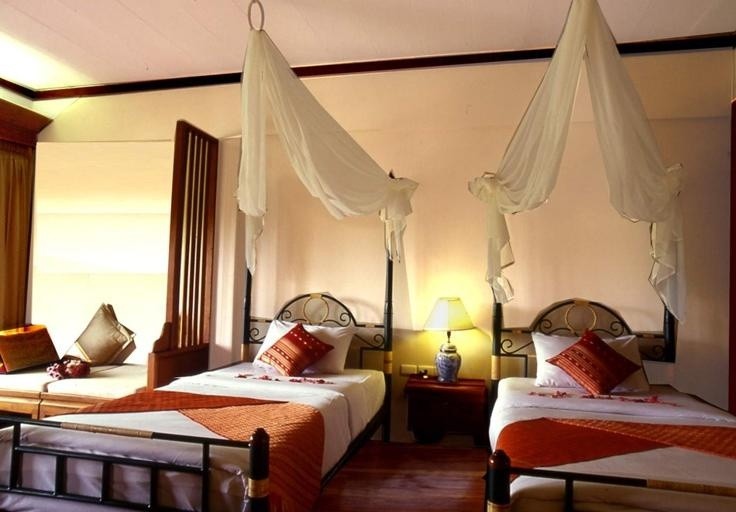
257;320;332;378
530;330;650;395
253;318;355;373
0;323;58;373
545;327;643;396
77;303;133;365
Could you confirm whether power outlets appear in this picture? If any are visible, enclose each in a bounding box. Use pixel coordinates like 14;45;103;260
416;365;437;377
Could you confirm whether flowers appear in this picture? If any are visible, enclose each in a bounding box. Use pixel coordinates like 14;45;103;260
45;356;91;382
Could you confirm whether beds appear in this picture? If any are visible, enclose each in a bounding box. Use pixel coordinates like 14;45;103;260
0;346;149;424
1;169;397;512
464;174;735;512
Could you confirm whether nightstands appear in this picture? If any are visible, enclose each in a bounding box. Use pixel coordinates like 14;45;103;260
403;373;487;447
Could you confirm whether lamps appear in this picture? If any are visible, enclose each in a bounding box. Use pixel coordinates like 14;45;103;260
424;293;476;384
